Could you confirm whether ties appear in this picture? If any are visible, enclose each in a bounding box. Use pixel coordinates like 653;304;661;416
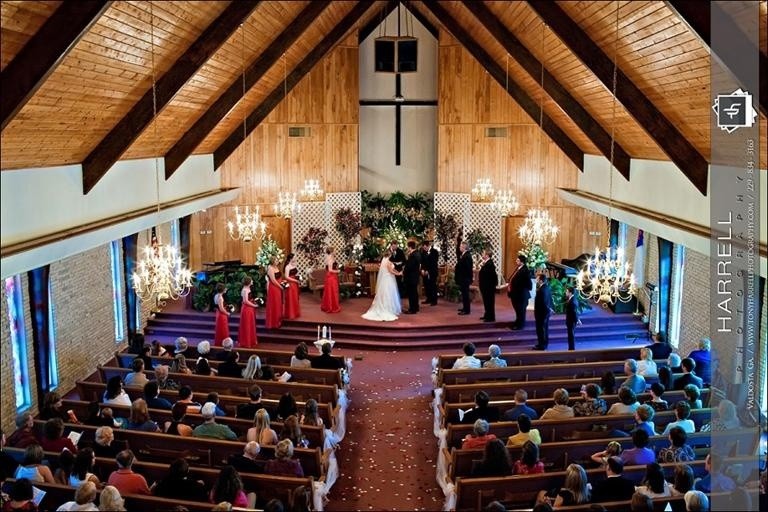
392;250;396;257
509;267;518;282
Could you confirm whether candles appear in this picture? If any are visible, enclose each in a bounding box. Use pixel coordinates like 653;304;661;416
329;327;332;342
317;325;320;342
322;324;327;338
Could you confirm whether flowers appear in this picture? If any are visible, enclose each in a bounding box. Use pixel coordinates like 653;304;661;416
255;232;286;267
519;246;549;278
334;189;463;303
295;226;334;267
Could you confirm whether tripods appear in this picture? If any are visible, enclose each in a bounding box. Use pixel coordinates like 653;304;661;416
632;290;664;343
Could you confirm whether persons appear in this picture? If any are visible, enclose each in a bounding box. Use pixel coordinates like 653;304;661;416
3;333;333;510
380;240;418;316
452;342;690;510
421;241;582;352
214;248;342;348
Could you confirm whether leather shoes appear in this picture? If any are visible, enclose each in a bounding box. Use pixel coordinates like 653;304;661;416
535;344;540;347
512;326;523;330
480;317;485;320
430;302;437;306
458;308;463;311
458;310;470;315
421;301;431;304
533;345;545;350
484;319;495;322
404;310;416;314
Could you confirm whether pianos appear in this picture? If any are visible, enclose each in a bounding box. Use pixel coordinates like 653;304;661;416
192;259;260;285
547;254;596;278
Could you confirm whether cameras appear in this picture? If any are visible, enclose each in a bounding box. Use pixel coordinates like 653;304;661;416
645;282;658;291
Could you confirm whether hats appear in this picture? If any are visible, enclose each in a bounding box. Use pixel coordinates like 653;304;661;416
201;402;216;417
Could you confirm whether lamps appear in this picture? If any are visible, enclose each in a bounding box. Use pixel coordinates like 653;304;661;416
131;0;194;302
301;179;324;201
515;22;560;249
490;53;520;218
624;282;659;343
473;177;494;202
273;53;301;220
574;1;639;310
226;23;267;243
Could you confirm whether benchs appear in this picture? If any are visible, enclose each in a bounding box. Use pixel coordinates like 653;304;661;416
0;343;352;512
308;270;356;298
430;348;768;511
